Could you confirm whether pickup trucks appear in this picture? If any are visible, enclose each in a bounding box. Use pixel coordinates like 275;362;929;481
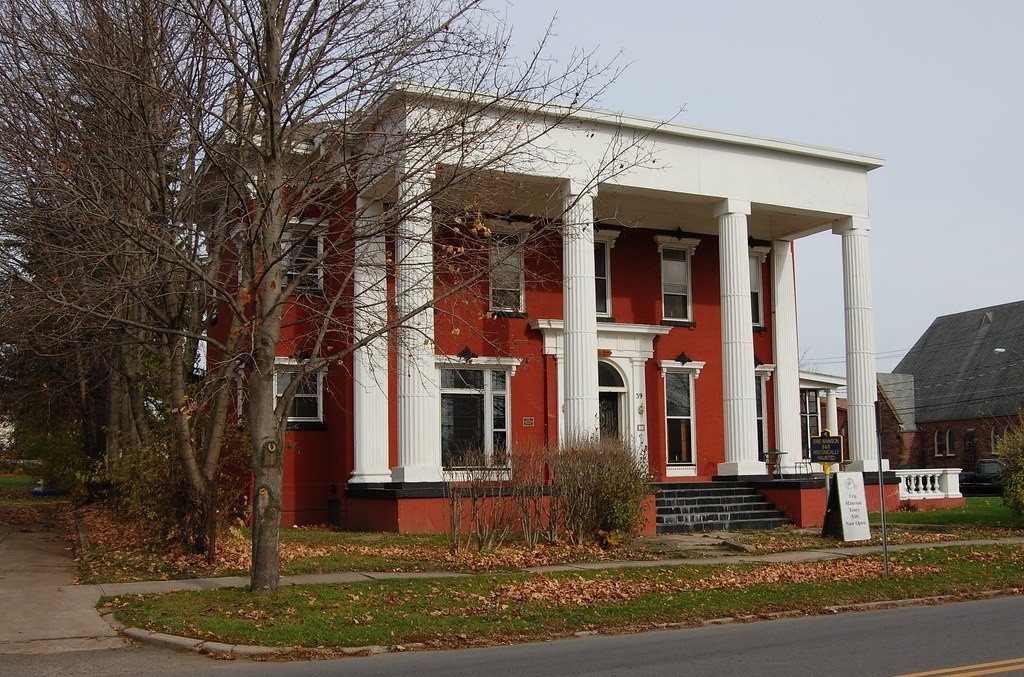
959;460;1012;494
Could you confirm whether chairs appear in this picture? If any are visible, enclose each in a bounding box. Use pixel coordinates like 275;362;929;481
766;449;784;480
795;447;814;479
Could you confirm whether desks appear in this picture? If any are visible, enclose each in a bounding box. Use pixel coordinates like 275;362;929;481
761;452;788;479
